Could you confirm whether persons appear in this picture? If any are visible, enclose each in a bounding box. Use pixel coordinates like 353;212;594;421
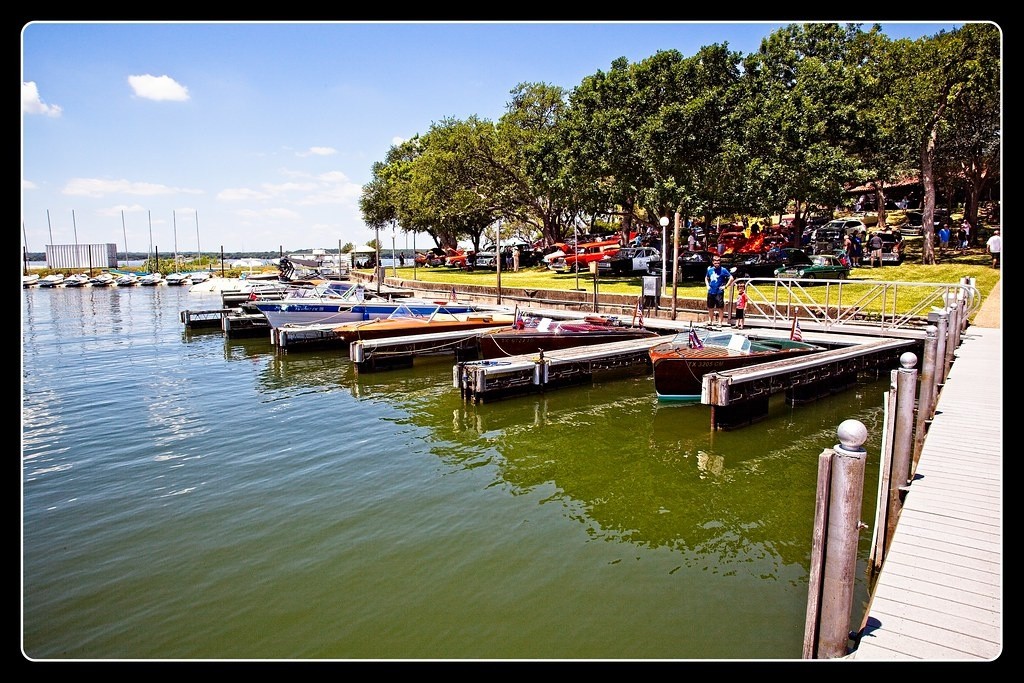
589;218;659;247
505;246;513;273
985;230;1000;269
954;219;971;255
869;232;884;268
512;246;520;273
425;251;432;268
902;195;908;214
687;233;696;251
399;252;405;268
731;283;748;330
938;222;950;254
704;256;734;328
843;228;867;267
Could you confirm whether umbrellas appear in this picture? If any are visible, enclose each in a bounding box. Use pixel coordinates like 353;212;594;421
348;245;376;266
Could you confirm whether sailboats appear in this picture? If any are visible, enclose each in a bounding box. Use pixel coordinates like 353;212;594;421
21;207;208;286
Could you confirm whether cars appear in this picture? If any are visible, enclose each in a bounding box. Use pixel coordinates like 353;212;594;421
414;220;907;288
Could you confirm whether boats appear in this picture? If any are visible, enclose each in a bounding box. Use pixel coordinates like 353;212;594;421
649;334;828;404
254;300;531;340
479;299;663;362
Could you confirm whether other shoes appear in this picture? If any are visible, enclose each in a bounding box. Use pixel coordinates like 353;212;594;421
739;327;743;330
717;324;722;328
732;326;738;328
